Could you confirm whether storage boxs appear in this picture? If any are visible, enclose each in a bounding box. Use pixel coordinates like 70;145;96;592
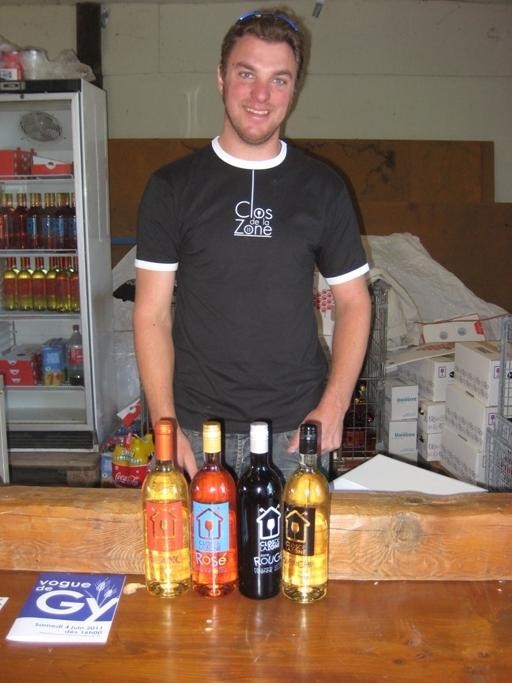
314;263;509;464
441;314;512;488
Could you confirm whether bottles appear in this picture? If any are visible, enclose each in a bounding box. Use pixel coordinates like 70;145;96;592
102;420;155;464
66;323;84;385
140;419;194;599
283;424;330;603
188;419;239;599
236;420;283;600
1;189;79;312
0;48;41;79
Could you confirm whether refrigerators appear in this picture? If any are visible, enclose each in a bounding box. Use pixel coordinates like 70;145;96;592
1;79;120;486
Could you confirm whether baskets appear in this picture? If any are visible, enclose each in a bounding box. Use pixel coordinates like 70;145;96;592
329;279;386;475
485;318;512;492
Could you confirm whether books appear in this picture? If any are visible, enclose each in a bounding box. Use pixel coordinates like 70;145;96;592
6;572;128;646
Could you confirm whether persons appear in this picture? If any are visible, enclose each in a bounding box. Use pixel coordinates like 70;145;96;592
133;7;372;486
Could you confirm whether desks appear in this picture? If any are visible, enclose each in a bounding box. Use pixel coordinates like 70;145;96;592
0;569;512;683
